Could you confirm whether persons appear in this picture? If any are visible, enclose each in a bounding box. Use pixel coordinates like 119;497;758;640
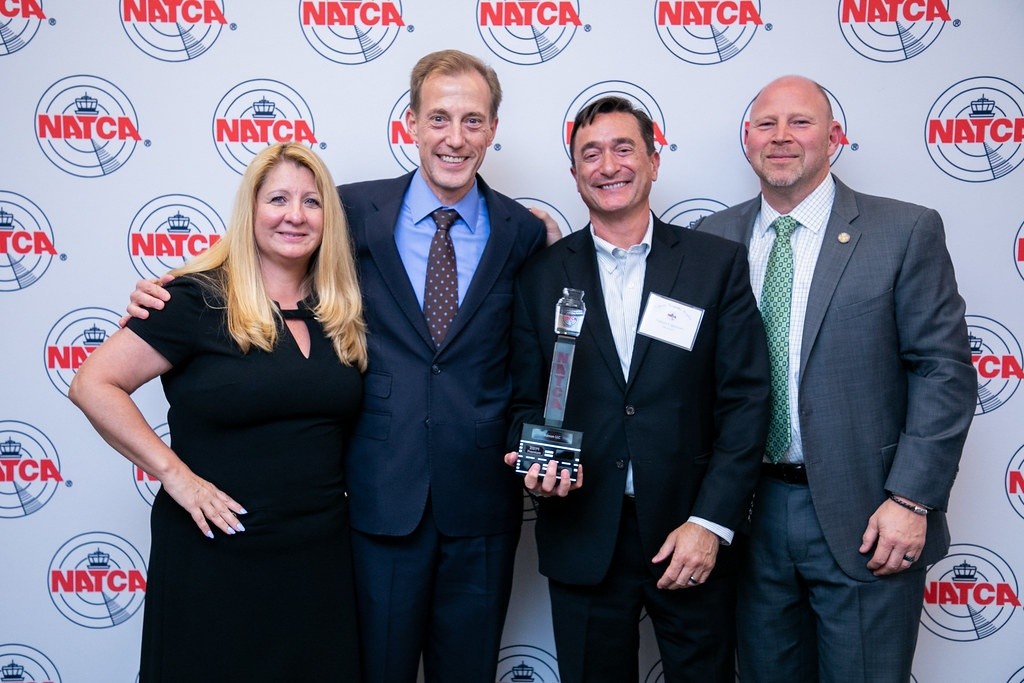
530;75;978;683
119;49;548;683
504;96;771;683
67;141;367;683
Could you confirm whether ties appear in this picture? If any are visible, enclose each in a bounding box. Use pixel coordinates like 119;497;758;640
759;216;802;464
422;209;460;348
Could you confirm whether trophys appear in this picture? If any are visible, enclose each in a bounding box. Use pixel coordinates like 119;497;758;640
514;287;586;482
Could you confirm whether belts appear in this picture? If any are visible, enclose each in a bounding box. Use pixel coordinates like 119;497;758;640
770;462;809;485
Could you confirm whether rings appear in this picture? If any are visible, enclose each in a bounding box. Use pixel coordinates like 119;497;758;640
689;577;697;584
903;555;915;562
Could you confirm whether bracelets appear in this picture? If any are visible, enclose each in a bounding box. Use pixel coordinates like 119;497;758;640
890;496;928;515
